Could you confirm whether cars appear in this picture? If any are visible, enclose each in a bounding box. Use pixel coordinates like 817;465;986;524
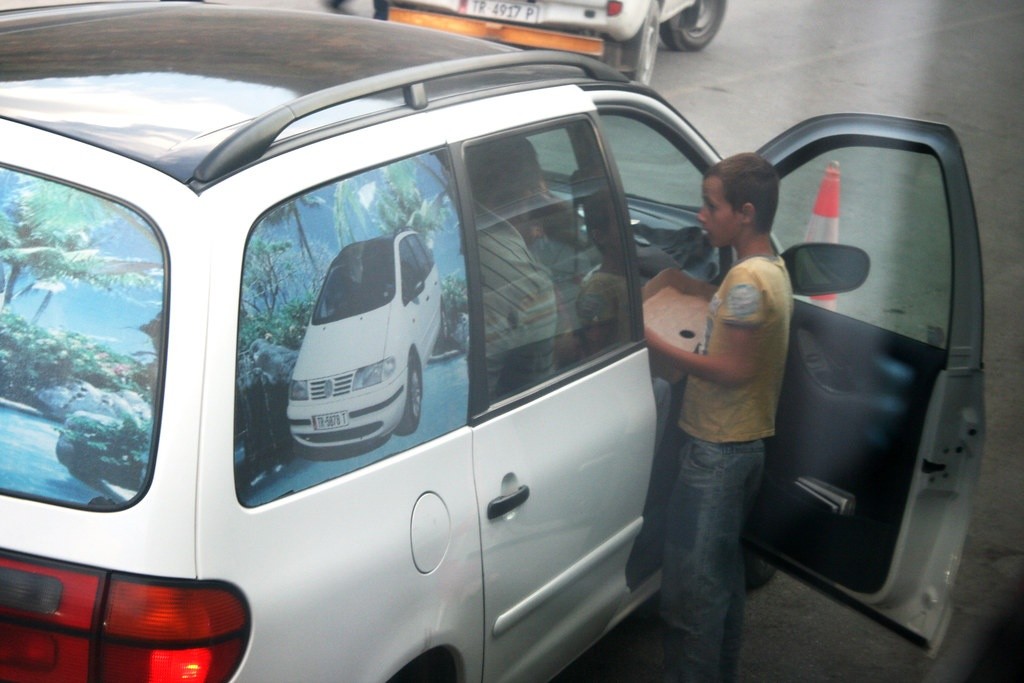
334;0;729;87
3;0;991;683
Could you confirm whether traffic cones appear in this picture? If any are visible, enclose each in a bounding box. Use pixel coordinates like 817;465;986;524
795;159;843;314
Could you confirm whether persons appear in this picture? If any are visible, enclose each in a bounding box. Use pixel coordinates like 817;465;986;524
467;133;600;406
644;153;793;683
